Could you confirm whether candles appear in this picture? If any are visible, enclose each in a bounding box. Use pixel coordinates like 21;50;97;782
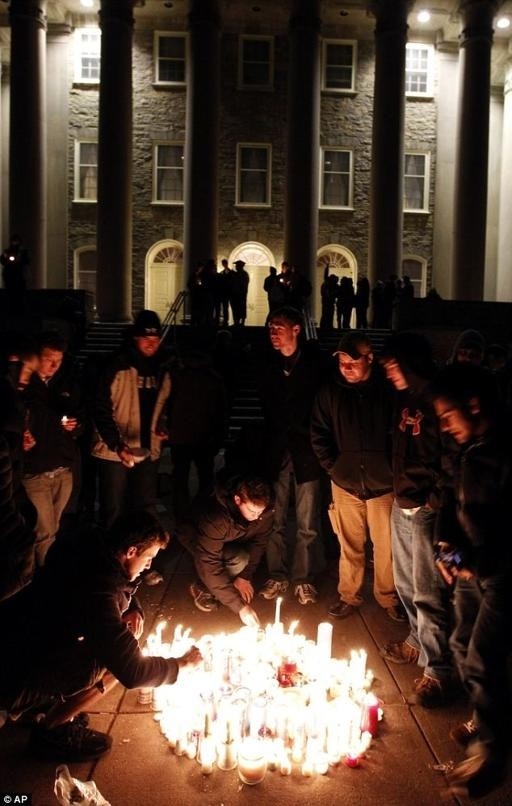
139;595;385;786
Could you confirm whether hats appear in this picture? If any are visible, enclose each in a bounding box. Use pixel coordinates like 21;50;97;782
332;333;372;360
134;310;161;337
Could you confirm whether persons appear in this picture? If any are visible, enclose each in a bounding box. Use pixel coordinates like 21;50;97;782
186;257;416;331
0;238;33;290
